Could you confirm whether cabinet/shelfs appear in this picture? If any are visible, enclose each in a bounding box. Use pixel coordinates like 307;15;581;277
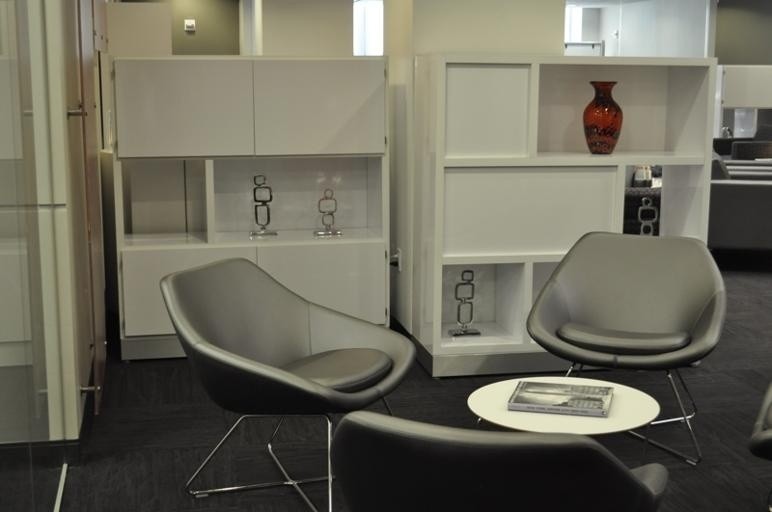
418;51;713;360
110;57;394;339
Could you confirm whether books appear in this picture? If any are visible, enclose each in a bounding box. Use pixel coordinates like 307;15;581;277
506;380;614;417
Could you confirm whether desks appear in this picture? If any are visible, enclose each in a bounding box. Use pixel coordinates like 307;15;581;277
467;377;660;434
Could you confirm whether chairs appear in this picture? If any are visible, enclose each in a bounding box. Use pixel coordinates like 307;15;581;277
525;232;728;464
331;410;669;511
713;141;771;253
160;256;418;511
